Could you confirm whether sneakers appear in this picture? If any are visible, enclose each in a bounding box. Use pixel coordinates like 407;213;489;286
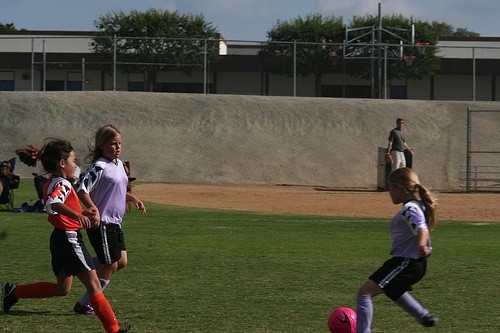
73;301;96;315
2;281;18;314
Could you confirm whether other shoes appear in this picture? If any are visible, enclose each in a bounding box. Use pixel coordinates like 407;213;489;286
420;314;439;327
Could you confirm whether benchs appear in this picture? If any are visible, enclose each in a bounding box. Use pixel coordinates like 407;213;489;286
459;163;500;191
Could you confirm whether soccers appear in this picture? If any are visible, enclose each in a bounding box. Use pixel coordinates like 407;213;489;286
328;307;359;333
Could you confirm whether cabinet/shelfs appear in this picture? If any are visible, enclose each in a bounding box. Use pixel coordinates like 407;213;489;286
378;145;412;190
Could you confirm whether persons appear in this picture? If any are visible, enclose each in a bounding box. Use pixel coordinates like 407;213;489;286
72;123;147;314
356;167;439;333
0;137;131;332
385;118;413;173
